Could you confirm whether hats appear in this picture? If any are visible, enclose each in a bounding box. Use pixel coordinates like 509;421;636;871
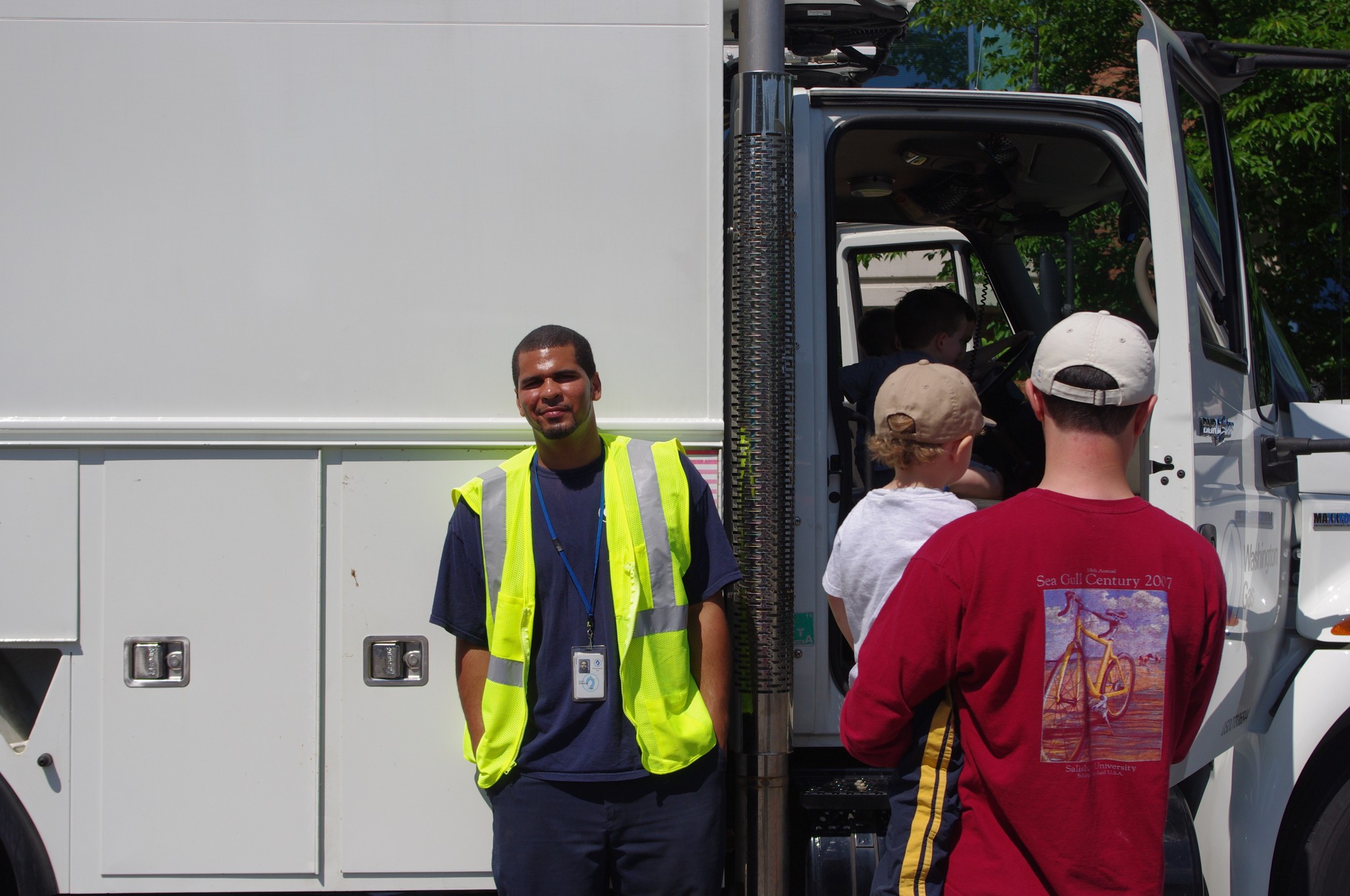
1030;307;1155;405
874;359;996;445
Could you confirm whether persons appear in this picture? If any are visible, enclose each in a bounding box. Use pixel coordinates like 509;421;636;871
430;325;741;896
840;309;1227;896
819;287;1005;896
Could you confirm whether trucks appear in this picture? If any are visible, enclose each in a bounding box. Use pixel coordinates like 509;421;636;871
2;0;1349;896
